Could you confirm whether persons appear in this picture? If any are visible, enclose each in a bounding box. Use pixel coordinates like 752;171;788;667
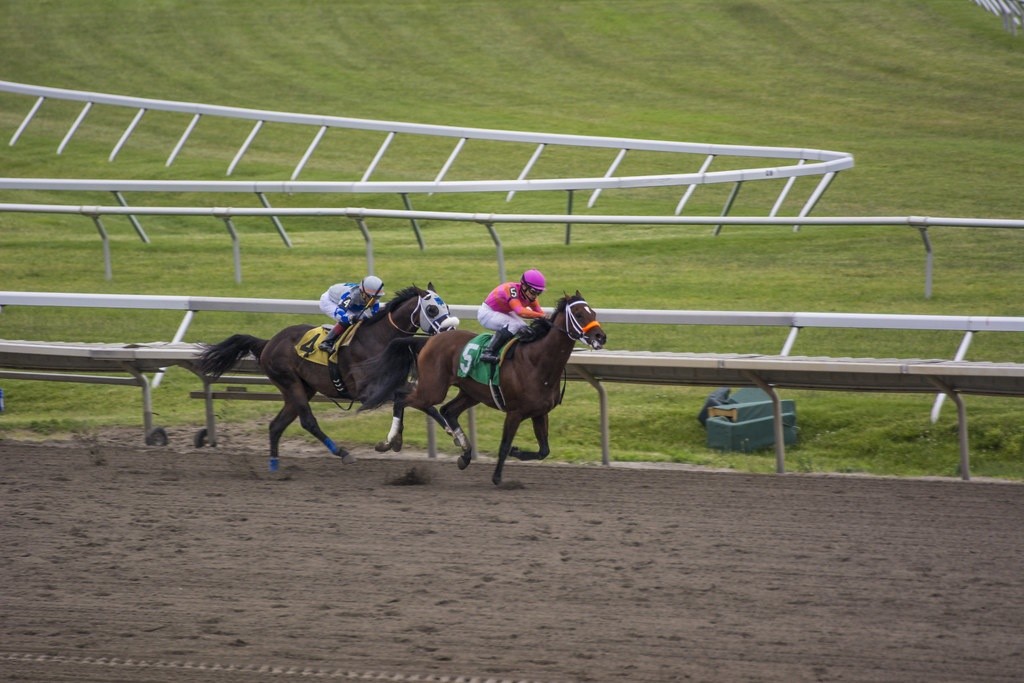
477;269;550;363
318;276;385;354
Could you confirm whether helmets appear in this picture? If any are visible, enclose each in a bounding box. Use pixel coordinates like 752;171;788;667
359;276;385;296
520;269;546;291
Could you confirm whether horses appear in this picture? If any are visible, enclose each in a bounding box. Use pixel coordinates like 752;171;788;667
194;281;457;473
352;289;607;486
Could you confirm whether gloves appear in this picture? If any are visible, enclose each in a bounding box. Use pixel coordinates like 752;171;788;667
352;315;359;324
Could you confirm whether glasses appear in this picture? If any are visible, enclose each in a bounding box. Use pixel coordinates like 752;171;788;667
522;274;542;296
362;280;381;300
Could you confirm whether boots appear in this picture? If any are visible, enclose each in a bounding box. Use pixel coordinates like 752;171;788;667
318;332;338;351
480;331;507;362
